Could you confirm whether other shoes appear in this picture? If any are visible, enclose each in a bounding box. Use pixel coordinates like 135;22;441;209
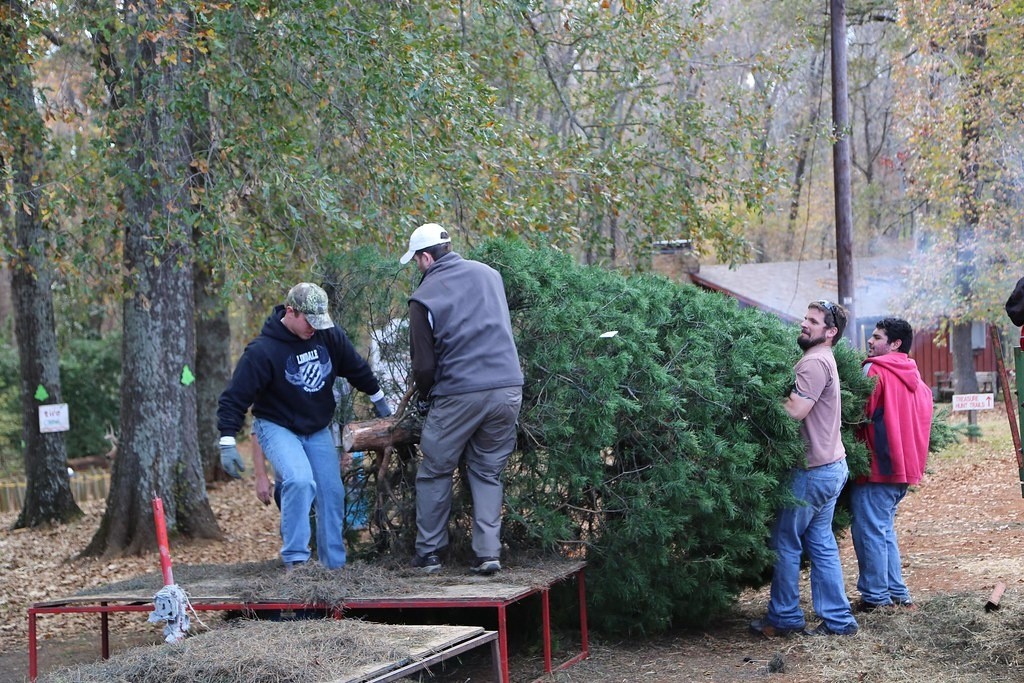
285;560;306;572
850;599;877;615
895;600;916;612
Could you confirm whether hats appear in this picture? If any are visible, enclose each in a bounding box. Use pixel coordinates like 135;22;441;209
400;223;451;264
287;282;334;329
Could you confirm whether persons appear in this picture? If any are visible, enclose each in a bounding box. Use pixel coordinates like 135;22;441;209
399;223;523;574
216;283;391;572
750;300;858;639
848;319;933;612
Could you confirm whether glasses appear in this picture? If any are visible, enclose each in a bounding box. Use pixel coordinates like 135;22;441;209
819;299;838;327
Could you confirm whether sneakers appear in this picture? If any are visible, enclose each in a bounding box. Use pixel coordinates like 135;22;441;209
750;616;806;639
411;552;441;573
470;556;501;574
805;619;858;636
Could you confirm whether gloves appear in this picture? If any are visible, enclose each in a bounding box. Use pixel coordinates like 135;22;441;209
373;396;391;418
417;396;430;417
219;445;245;479
